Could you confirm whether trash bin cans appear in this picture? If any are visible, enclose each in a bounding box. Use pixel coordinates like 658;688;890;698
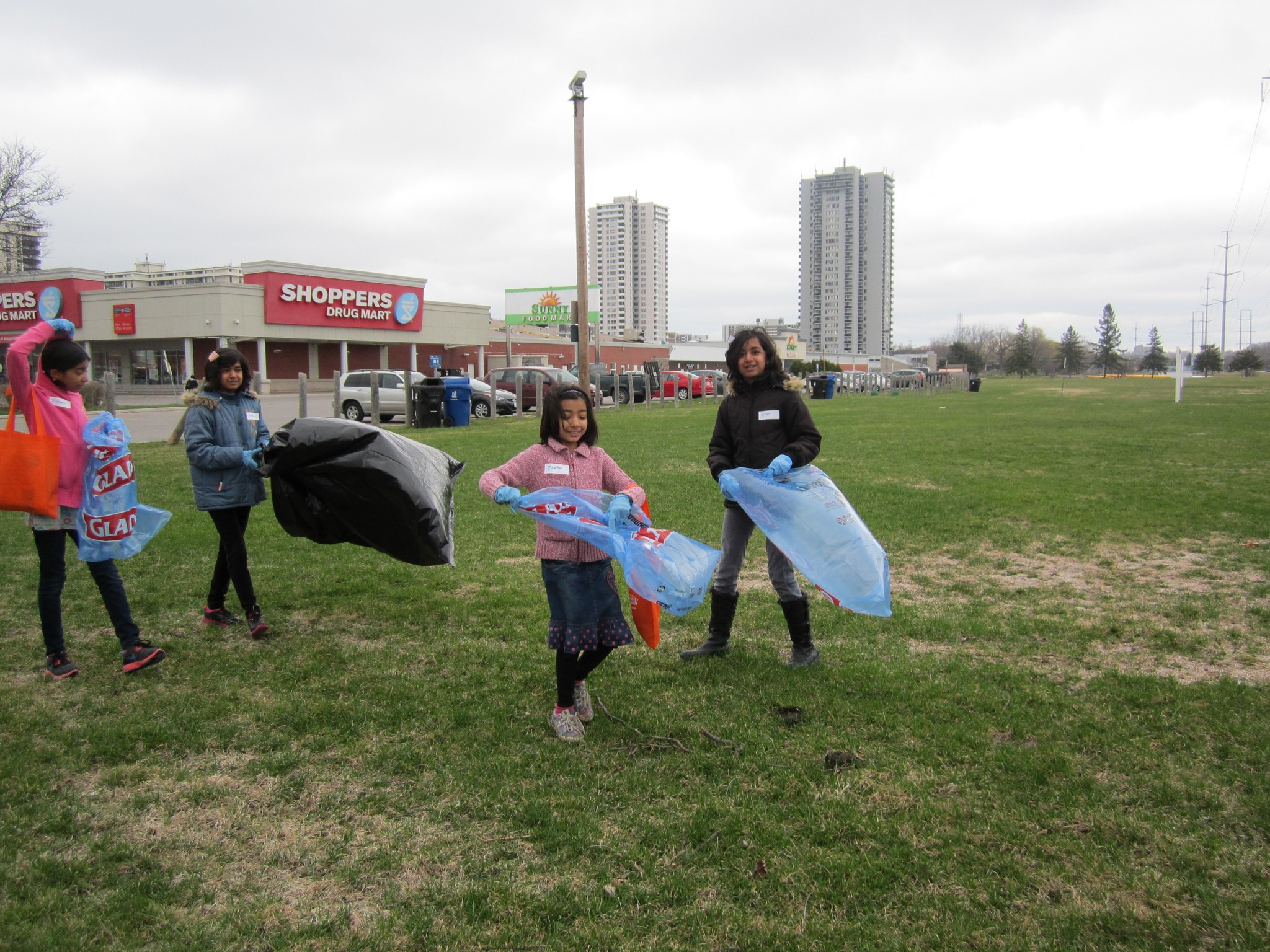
825;374;836;399
411;377;447;429
442;375;474;427
970;378;982;392
809;375;829;399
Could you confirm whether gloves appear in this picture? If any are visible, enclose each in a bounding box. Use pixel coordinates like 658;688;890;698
606;493;632;532
258;440;270;452
494;486;521;514
718;473;741;502
242;448;262;470
45;318;75;339
764;454;792;481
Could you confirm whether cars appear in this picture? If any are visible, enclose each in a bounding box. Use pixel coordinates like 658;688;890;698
623;371;645;375
689;370;731;395
439;377;519;417
802;369;895;394
650;370;714;400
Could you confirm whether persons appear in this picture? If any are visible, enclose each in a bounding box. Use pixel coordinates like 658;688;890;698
186;375;198;392
675;326;825;673
181;348;273;640
7;319;165;676
479;384;649;742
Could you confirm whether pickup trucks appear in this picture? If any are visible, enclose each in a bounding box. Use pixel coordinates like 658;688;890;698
911;366;954;386
569;362;662;404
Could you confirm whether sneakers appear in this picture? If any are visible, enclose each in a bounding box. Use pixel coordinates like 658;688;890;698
572;681;594;722
247;612;269;637
45;652;79;680
123;643;166;674
551;708;584;742
202;605;244;628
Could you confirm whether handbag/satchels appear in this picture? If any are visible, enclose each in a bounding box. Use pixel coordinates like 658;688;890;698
0;382;60;520
626;484;661;650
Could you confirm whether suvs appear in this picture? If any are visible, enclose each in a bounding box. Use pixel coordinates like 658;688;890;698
887;369;928;390
331;368;445;422
482;366;604;407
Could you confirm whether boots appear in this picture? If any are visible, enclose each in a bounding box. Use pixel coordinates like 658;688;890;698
777;592;822;669
680;586;739;661
